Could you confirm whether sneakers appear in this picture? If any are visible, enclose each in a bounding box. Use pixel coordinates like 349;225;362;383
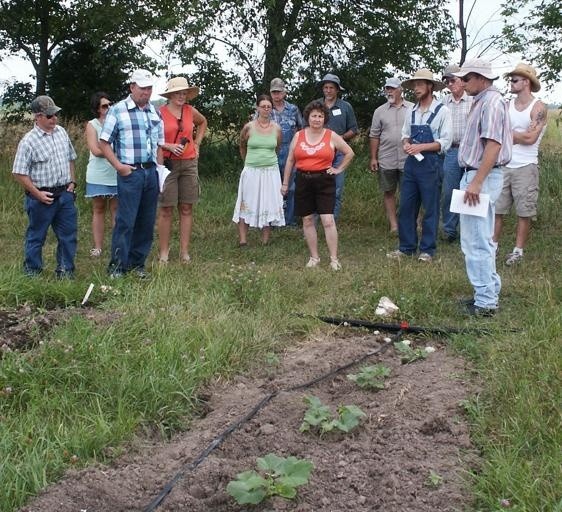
307;257;320;267
331;259;342;271
505;251;522;267
387;251;408;259
90;249;102;255
108;266;149;281
463;299;495;318
419;252;433;263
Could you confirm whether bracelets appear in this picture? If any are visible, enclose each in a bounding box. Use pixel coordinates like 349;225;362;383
70;181;77;188
283;184;288;186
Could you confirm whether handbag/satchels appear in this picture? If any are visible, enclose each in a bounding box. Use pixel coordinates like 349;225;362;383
164;156;173;170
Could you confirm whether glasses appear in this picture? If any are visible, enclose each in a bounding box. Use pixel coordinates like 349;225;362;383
99;102;112;110
40;113;57;118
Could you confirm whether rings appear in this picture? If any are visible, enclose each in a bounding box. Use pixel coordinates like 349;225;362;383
175;150;177;151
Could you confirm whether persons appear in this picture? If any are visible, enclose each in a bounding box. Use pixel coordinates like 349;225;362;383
440;64;473;243
451;57;513;319
385;68;454;264
369;76;423;232
281;100;355;270
85;92;118;255
253;77;305;231
232;95;286;249
99;68;164;281
158;76;207;264
12;95;78;281
301;74;359;231
492;63;547;265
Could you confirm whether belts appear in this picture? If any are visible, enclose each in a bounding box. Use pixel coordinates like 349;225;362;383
128;163;153;171
459;166;498;172
40;185;67;192
451;143;460;147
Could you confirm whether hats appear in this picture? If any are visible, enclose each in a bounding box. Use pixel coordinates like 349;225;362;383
31;96;62;114
401;70;446;91
270;78;285;91
503;63;540;92
442;65;459;80
317;73;346;91
452;58;499;80
159;77;200;99
131;70;154;88
384;78;402;88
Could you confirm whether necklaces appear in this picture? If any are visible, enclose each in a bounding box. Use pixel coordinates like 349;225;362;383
257;118;270;128
